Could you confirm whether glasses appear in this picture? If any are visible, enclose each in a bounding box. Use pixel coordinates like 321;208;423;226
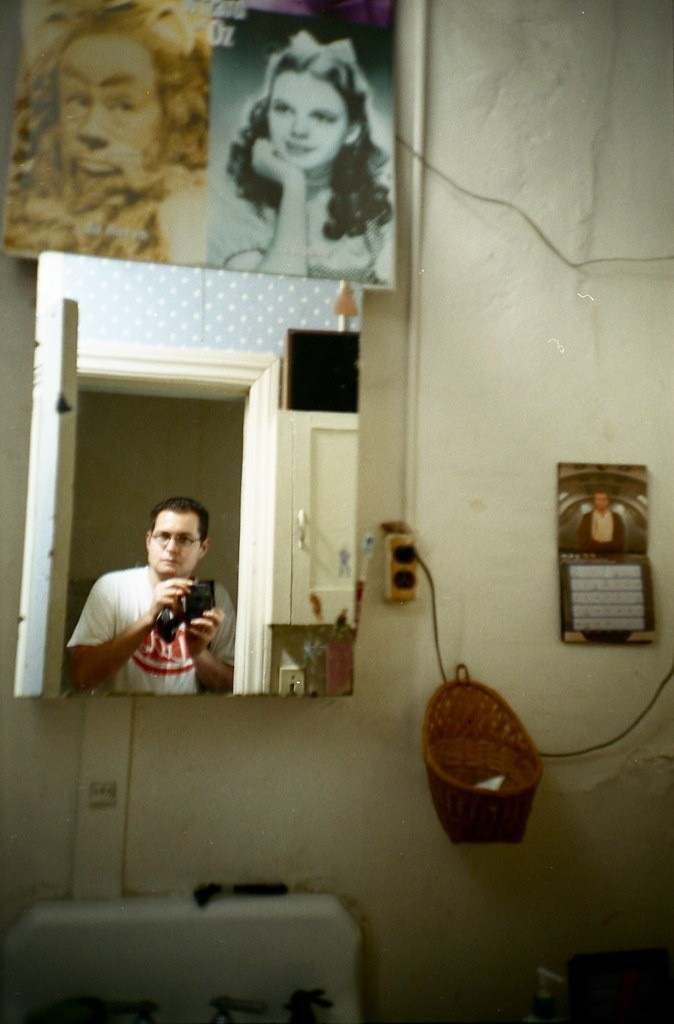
150;532;203;547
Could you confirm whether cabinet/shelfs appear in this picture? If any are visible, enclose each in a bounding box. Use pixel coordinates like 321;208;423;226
264;410;358;629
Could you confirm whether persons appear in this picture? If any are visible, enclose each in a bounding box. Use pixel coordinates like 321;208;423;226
576;489;626;553
0;0;209;267
205;31;394;284
66;496;237;693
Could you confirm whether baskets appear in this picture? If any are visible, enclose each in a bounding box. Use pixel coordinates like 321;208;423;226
422;664;543;846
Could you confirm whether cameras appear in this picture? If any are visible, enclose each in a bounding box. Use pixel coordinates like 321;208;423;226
183;585;211;624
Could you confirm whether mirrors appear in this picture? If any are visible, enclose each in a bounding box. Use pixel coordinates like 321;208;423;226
11;246;369;711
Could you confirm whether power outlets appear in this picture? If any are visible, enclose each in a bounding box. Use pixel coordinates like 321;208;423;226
384;533;419;603
